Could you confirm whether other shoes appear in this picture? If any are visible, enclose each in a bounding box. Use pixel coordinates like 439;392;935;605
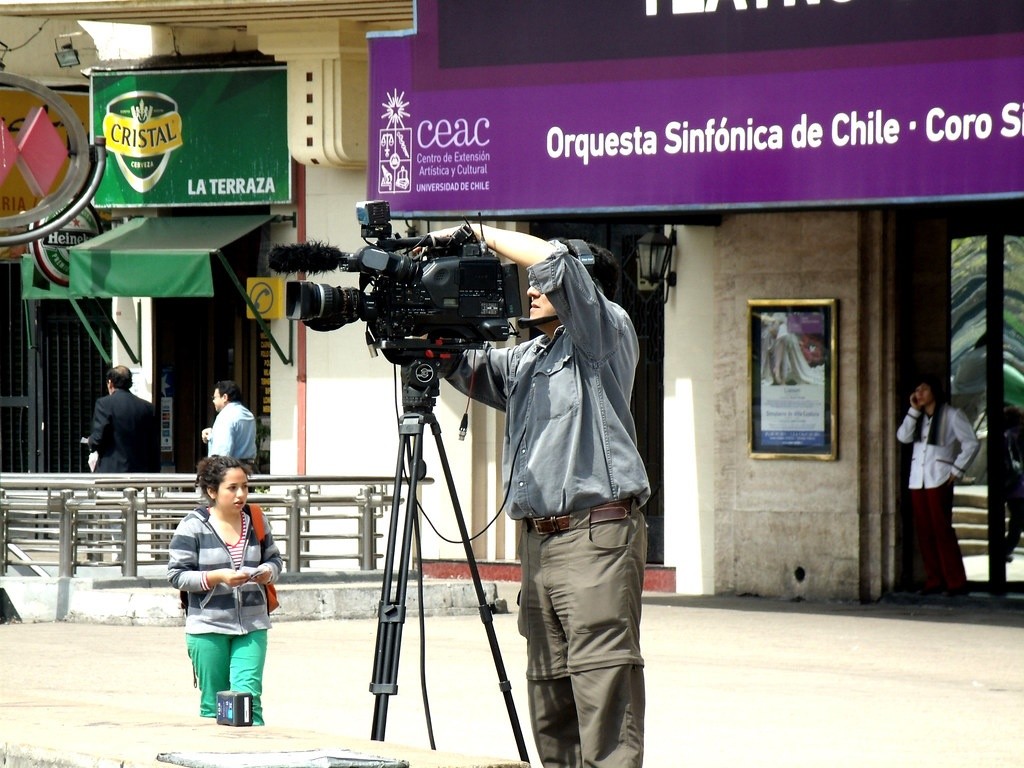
941;584;973;596
1005;556;1012;562
916;586;943;596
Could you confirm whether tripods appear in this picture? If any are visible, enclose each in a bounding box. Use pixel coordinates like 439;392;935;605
368;339;530;764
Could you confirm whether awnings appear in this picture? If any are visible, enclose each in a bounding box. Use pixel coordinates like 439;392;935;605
21;212;297;364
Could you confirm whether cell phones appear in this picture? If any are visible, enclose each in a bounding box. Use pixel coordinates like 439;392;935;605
912;397;917;404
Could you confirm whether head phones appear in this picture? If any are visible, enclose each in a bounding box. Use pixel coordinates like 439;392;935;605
566;238;604;295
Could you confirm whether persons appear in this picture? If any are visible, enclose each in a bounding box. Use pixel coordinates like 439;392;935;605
897;376;980;596
166;454;283;726
424;223;651;768
1003;405;1024;564
88;365;162;561
202;380;260;494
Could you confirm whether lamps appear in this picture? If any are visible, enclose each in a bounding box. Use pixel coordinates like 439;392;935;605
636;224;677;291
55;35;80;69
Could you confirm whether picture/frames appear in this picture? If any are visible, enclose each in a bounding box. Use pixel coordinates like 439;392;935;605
745;298;836;462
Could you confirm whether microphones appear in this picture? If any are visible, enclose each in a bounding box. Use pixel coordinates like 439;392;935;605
518;315;559;329
268;242;344;274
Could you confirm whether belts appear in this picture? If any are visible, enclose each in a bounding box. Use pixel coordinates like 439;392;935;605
527;500;632;534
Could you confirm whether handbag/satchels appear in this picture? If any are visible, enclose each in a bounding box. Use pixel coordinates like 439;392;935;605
1005;466;1020;494
266;584;279;613
